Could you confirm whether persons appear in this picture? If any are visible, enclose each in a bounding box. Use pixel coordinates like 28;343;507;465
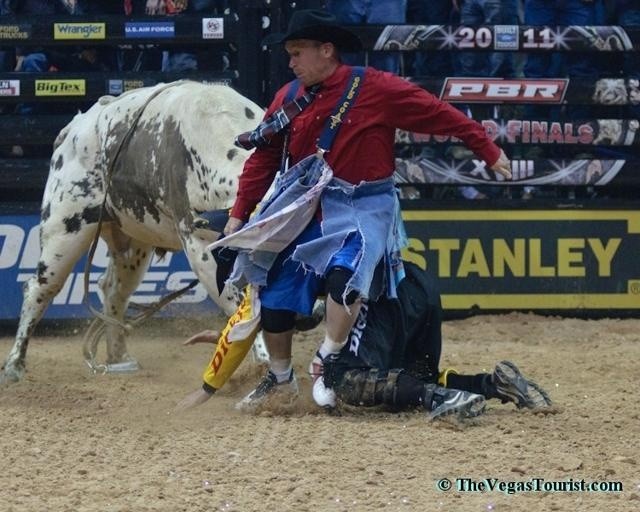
222;7;514;414
1;0;640;199
183;245;552;424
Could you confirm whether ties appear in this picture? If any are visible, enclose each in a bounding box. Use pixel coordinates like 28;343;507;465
234;83;322;151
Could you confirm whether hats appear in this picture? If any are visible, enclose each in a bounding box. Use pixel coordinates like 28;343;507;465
283;10;353;57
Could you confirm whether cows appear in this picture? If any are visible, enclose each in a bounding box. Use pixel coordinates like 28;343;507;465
0;79;328;389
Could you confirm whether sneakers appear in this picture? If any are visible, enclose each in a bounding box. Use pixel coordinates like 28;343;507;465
426;390;486;423
310;351;338;410
234;369;299;412
490;361;551;413
